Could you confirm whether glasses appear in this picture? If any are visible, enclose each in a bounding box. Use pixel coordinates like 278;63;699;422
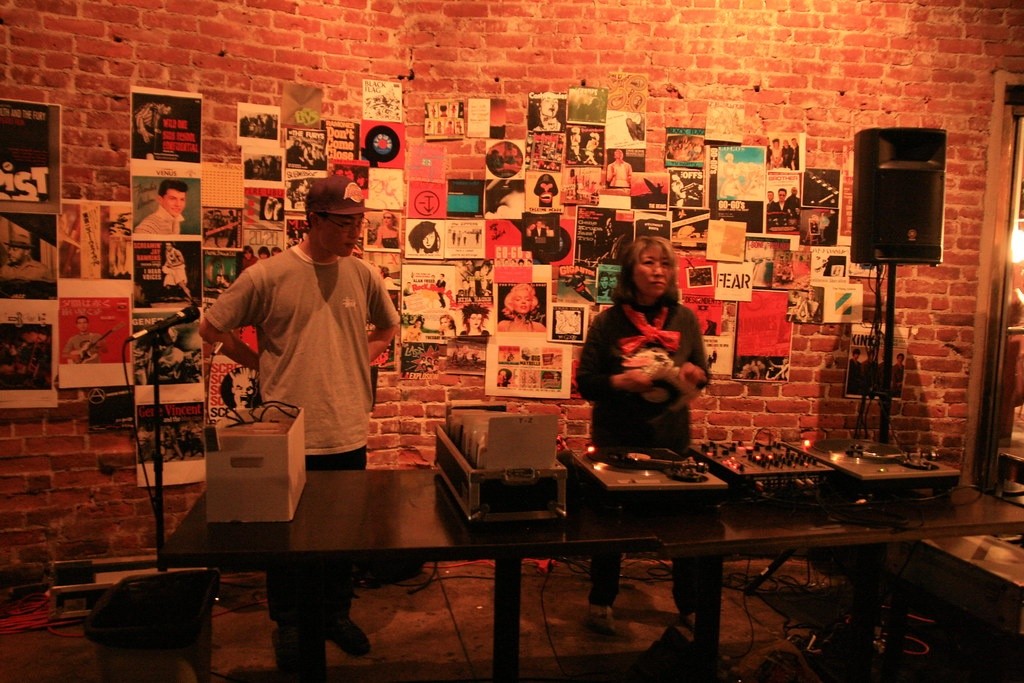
383;216;392;219
327;217;370;230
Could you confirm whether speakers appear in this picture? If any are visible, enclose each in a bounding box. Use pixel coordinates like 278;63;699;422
850;127;948;268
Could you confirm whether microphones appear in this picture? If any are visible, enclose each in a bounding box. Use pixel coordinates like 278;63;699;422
126;306;201;344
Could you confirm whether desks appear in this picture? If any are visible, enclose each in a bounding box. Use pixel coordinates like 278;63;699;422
160;458;1023;681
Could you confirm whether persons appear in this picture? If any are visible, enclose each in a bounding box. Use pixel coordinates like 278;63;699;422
135;103;171;159
405;318;425;342
607;150;631;187
766;137;801;171
198;175;400;683
378;212;399;249
162;242;195;303
497;285;545;333
849;349;904;396
2;235;50;283
135;179;187;236
789;289;821;322
766;187;800;232
243;245;282;268
64;315;115;363
459;303;490;336
440;314;455;336
577;235;732;642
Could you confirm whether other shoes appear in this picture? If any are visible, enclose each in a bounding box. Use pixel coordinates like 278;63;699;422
330;615;371;657
586;604;618;638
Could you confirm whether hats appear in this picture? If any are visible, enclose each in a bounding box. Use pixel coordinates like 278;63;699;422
304;174;368;215
